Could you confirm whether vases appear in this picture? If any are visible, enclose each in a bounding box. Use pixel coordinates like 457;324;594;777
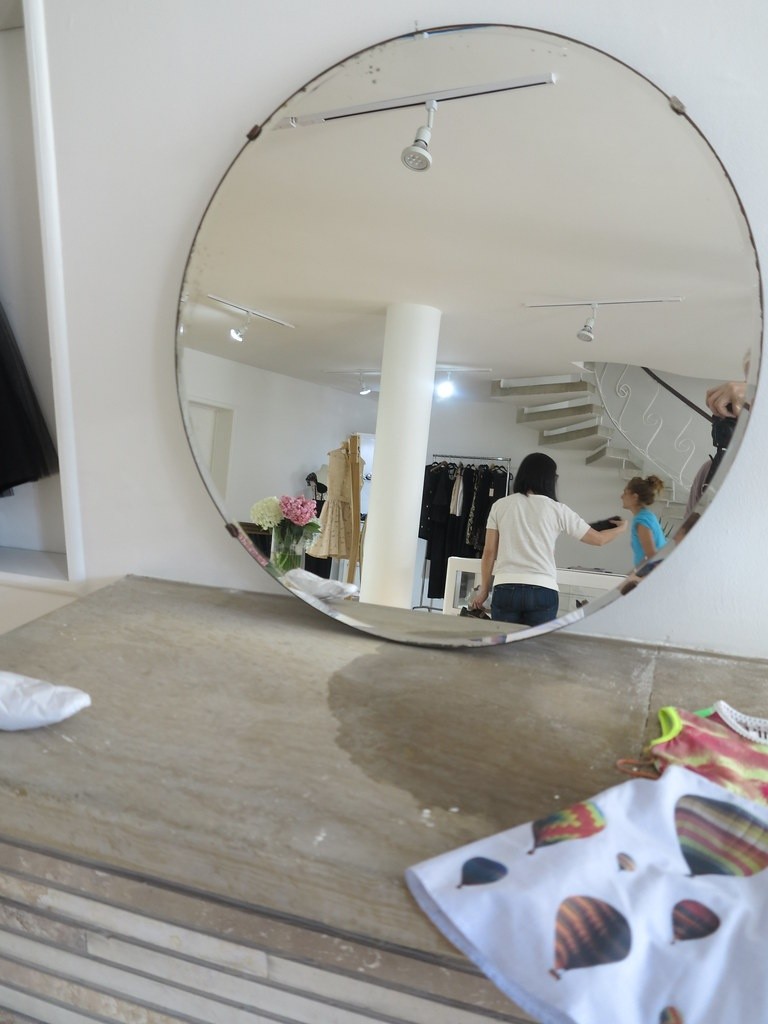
269;520;308;573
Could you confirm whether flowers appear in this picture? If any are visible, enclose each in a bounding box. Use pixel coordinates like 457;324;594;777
251;496;323;568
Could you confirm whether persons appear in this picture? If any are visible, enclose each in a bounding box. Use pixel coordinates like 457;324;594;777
704;380;748;423
621;475;666;568
470;451;628;631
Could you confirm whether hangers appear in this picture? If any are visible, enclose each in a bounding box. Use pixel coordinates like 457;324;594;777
429;454;514;482
326;439;359;457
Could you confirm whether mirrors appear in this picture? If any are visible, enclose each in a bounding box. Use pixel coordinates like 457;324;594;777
171;25;765;649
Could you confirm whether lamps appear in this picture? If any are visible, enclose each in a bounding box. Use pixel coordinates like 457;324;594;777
401;99;437;173
576;304;597;342
229;311;252;343
359;376;371;395
